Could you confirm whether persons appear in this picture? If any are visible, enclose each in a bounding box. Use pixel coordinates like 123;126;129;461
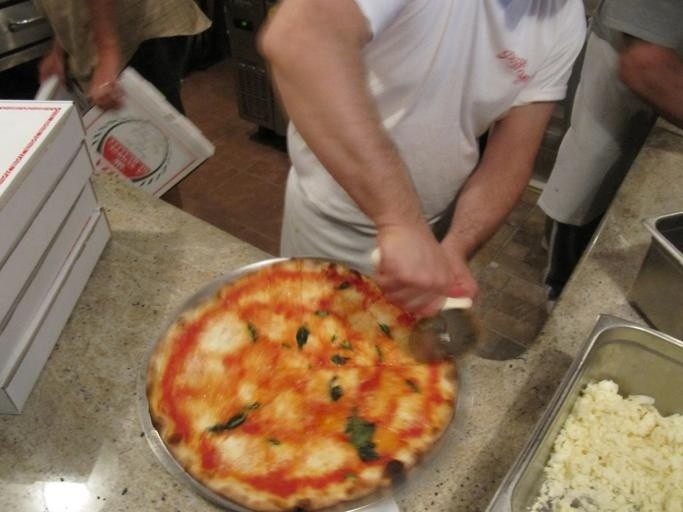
31;0;212;118
536;0;683;315
255;0;587;321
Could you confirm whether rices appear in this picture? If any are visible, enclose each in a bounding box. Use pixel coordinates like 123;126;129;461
525;380;683;512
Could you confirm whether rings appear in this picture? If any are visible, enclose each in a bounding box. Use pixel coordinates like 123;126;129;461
98;81;111;88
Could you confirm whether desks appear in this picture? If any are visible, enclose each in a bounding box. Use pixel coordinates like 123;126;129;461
0;114;682;510
